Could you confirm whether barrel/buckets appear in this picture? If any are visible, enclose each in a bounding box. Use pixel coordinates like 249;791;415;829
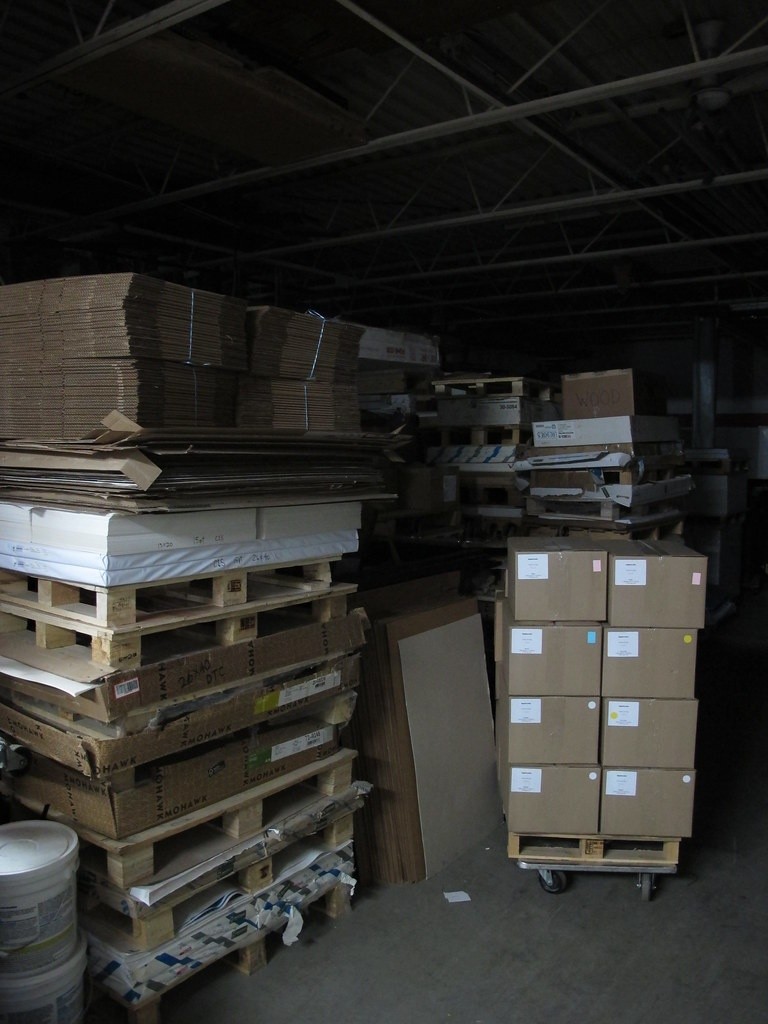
0;820;89;1024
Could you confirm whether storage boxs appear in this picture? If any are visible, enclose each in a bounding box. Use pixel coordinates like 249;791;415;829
350;316;441;371
400;464;445;512
560;366;668;419
485;534;709;838
682;469;749;518
687;523;748;597
